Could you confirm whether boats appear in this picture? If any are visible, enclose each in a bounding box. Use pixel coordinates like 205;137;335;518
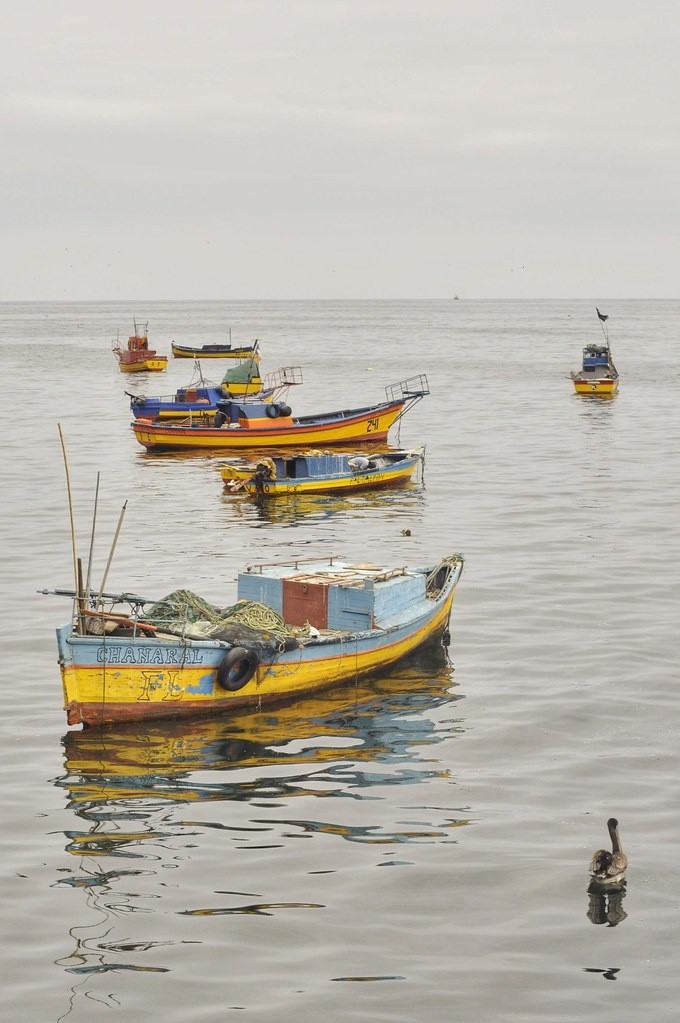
219;444;427;498
569;306;620;394
36;430;462;727
123;337;432;452
169;325;259;359
111;314;169;373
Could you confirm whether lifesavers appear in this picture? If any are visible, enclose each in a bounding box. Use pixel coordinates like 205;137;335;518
265;403;280;418
216;645;260;692
226;392;235;399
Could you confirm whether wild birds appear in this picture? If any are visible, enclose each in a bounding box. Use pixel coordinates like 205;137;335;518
589;818;628;884
